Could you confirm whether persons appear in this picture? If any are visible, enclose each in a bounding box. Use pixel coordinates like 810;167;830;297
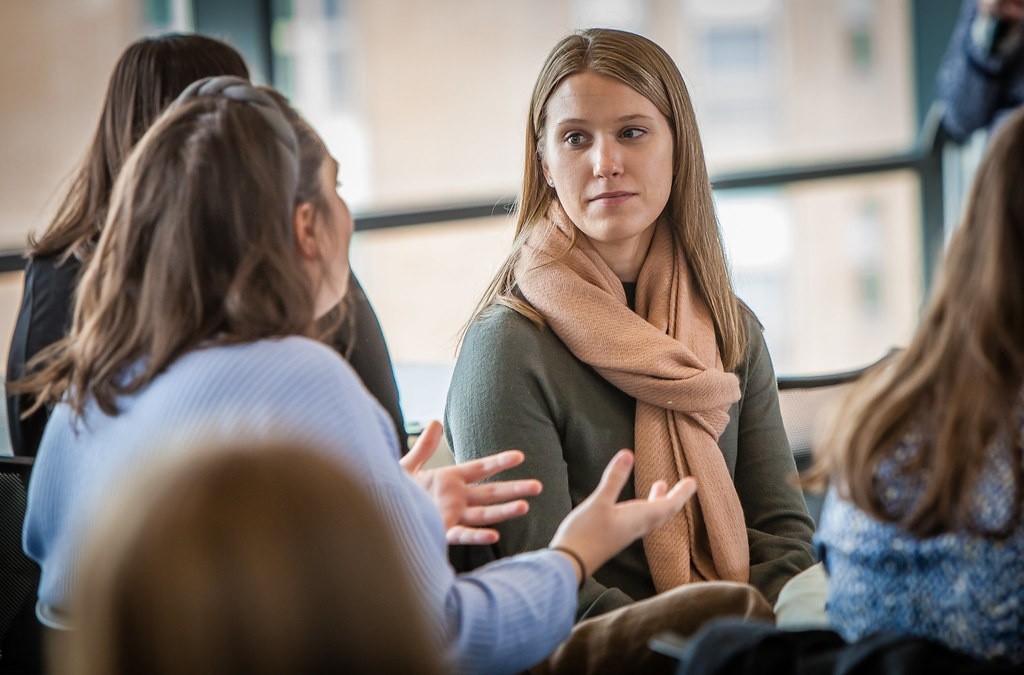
938;2;1024;143
43;423;460;673
7;77;700;675
11;31;412;458
816;104;1022;669
445;24;819;630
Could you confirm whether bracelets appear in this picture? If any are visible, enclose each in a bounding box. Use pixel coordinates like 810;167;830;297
549;545;588;591
